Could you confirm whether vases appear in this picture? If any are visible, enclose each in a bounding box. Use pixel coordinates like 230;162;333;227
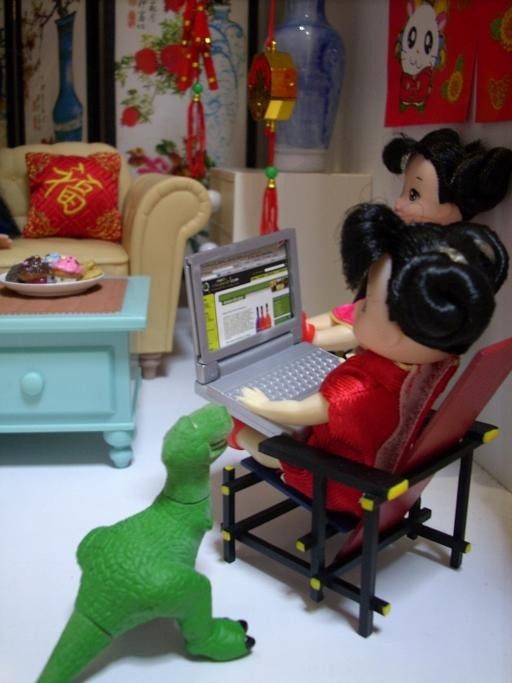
260;1;346;172
52;10;83;143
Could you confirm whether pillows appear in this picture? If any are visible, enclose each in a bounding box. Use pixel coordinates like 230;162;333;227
23;150;124;242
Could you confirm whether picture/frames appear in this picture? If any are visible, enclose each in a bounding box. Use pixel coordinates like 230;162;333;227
3;0;102;149
103;0;260;189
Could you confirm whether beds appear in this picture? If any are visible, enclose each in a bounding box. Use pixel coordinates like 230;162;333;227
0;276;150;469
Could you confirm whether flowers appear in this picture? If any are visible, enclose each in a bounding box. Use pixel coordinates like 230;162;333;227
118;8;240;179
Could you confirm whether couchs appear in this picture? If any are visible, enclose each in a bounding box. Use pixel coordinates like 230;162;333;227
0;141;212;379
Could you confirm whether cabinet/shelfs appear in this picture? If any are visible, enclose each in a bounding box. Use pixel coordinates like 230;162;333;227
208;167;372;319
